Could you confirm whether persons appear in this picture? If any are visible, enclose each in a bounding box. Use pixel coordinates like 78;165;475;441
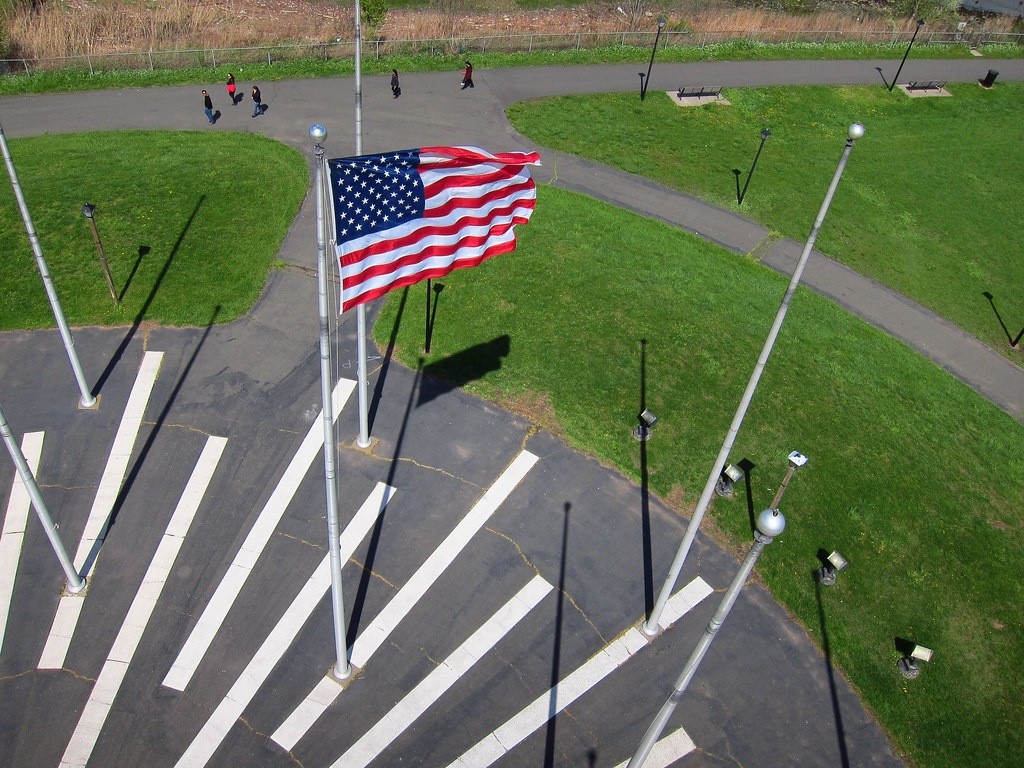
227;74;237;105
202;90;215;124
252;86;264;117
391;69;399;98
461;61;474;90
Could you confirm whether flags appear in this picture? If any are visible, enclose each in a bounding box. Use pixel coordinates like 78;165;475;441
327;145;542;313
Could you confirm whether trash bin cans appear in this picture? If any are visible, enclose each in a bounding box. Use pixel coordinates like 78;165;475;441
982;69;999;88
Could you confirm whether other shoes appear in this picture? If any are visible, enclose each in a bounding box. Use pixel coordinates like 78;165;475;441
461;87;465;90
231;102;238;106
208;120;214;125
393;93;398;99
470;86;474;88
252;111;262;118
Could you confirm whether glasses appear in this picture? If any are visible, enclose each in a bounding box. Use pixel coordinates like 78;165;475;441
203;93;206;94
